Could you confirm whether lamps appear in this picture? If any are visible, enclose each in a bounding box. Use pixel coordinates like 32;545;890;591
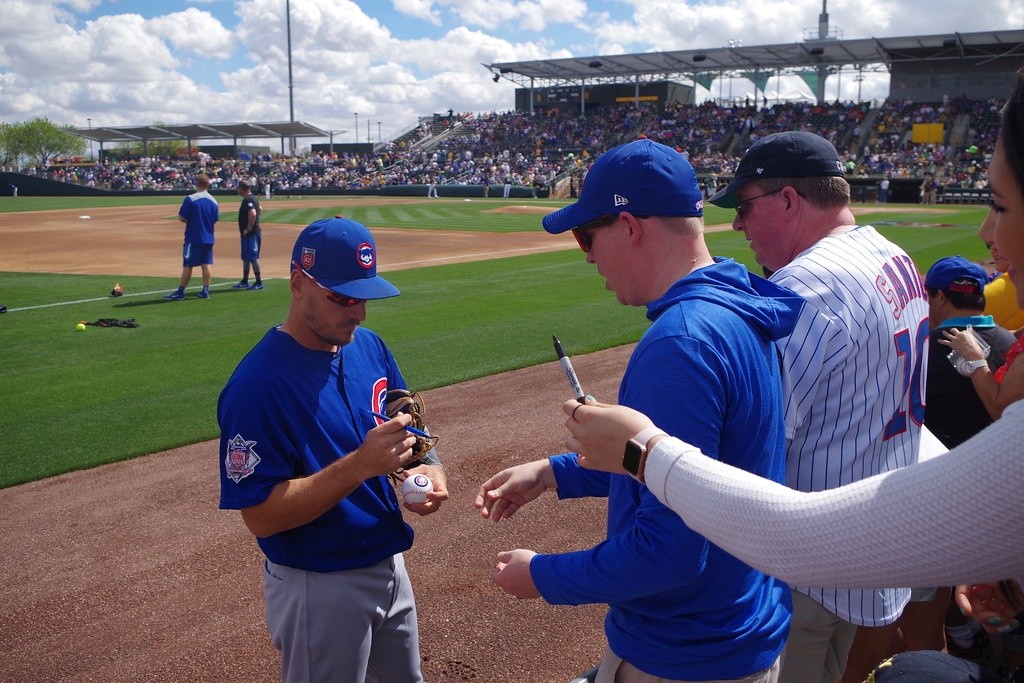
693;55;706;61
493;73;499;82
500;67;513;73
590;61;601;68
943;40;957;46
810;48;824;55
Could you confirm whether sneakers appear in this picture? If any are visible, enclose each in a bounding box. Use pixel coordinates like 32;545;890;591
198;292;210;298
247;284;265;289
232;283;250;288
163;292;185;300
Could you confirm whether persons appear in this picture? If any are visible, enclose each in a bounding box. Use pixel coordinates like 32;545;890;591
231;181;265;291
162;175;219;302
45;107;694;198
474;136;795;683
215;217;451;683
474;85;1023;682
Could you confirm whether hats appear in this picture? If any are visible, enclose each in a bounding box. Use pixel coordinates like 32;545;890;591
542;135;704;234
707;130;845;209
924;255;985;292
290;216;401;300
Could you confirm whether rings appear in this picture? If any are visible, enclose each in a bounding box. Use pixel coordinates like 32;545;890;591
572;404;583;422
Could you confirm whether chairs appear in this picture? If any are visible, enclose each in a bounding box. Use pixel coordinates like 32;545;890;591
944;192;992;204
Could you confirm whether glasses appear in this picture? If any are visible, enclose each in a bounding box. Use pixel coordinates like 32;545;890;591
292;260;365;306
734;187;806;219
571;213;649;253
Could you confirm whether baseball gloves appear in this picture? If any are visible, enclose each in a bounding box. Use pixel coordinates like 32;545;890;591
386;388;433;470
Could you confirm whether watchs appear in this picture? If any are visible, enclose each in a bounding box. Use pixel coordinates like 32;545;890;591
622;427;670;484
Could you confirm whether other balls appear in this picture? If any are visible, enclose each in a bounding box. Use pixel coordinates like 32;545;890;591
76;323;86;330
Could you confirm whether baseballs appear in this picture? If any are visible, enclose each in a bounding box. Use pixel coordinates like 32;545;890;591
401;473;434;506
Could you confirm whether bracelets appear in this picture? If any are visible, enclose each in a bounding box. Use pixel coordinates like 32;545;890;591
959;355;989;374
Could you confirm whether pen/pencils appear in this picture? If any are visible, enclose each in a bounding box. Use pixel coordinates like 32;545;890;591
366;409;430;441
551;334;587;405
